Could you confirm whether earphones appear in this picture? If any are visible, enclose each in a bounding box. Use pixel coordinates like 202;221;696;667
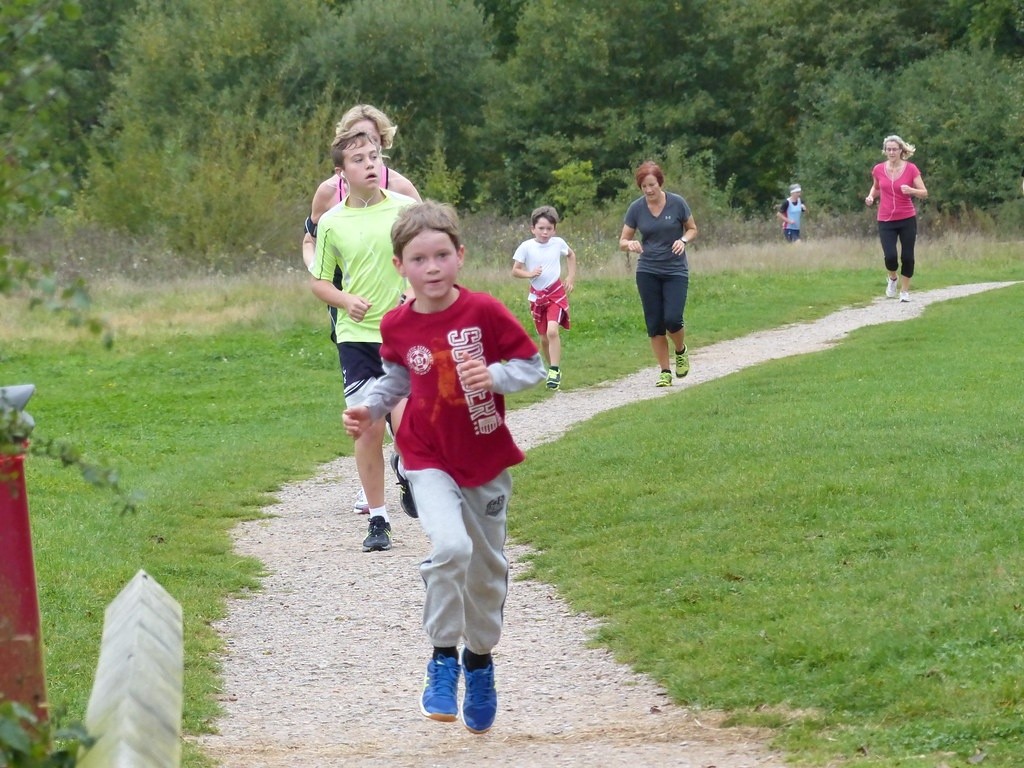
341;172;346;181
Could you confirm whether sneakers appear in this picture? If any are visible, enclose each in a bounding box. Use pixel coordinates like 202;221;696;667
886;273;899;297
419;655;460;722
362;516;391;552
392;452;419;519
657;373;673;388
353;488;371;515
674;346;689;378
900;291;909;304
546;366;562;390
461;648;498;735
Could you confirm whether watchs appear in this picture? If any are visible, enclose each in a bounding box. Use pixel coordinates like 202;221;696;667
679;237;687;245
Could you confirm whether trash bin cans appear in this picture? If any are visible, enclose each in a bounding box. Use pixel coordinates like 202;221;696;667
0;384;54;754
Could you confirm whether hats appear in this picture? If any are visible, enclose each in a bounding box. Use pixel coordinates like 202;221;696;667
789;183;801;194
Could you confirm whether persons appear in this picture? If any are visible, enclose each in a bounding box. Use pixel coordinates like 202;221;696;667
619;163;697;388
304;105;424;516
866;136;928;303
513;206;575;391
341;204;547;734
309;128;420;552
777;183;806;243
